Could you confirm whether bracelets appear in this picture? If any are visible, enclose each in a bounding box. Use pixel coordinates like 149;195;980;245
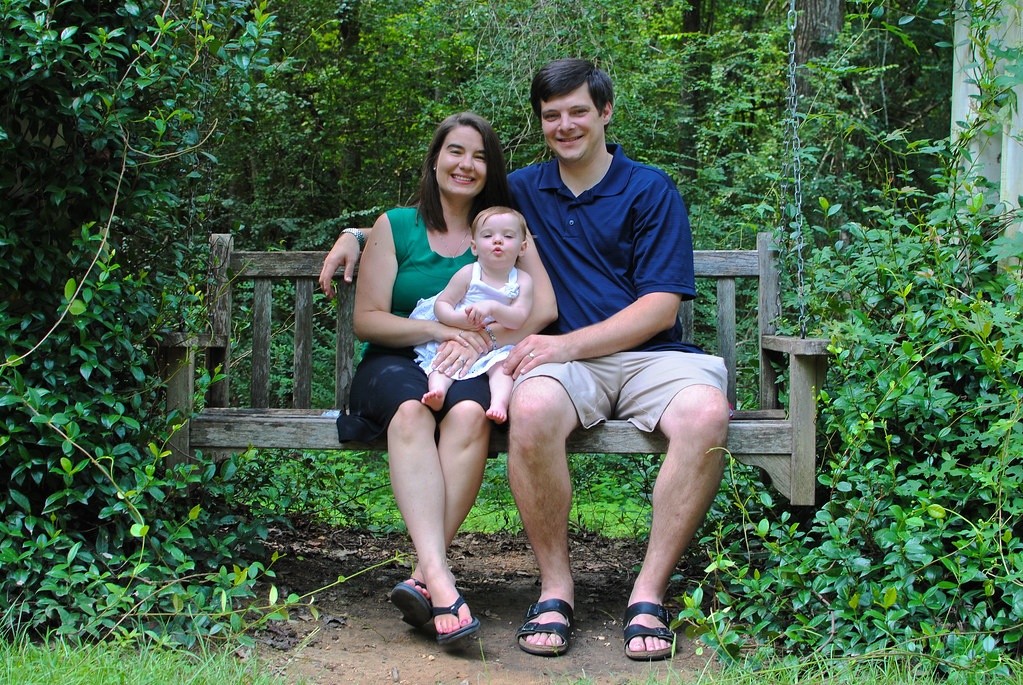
339;228;366;251
485;326;496;350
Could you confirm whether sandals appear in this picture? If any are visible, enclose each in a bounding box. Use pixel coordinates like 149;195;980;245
622;602;674;659
514;599;573;658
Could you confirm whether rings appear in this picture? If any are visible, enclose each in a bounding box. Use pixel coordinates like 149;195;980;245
458;358;466;364
459;330;464;336
528;353;534;358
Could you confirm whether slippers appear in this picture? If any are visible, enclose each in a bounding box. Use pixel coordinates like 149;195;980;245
431;595;480;644
391;577;433;627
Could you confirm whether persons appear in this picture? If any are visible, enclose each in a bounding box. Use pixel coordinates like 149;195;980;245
408;206;535;422
337;111;559;645
319;59;733;660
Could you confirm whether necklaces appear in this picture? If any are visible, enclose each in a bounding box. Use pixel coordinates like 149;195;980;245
434;228;469;258
